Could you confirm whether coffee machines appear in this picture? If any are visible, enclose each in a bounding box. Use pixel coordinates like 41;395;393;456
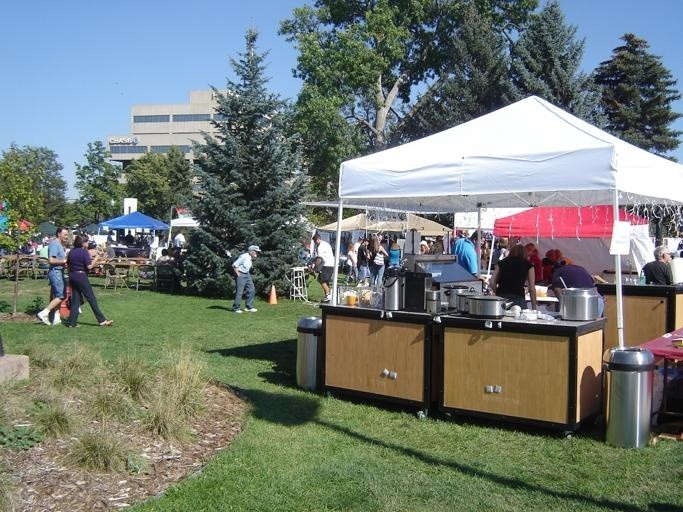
383;267;432;312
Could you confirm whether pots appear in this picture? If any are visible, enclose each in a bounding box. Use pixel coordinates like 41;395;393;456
559;286;600;321
442;284;508;319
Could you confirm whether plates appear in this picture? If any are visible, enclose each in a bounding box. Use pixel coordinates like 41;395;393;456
506;309;546;321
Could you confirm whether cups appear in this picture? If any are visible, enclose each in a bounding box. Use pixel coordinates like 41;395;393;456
339;275;383;308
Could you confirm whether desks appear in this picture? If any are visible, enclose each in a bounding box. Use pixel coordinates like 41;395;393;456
635;328;683;438
431;311;608;440
594;283;683;352
318;303;458;419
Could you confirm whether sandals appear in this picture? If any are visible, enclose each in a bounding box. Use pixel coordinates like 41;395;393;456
98;319;113;326
68;324;80;327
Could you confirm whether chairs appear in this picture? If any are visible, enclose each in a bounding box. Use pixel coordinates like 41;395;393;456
0;247;189;291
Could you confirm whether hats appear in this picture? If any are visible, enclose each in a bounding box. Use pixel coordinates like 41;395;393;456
247;245;261;253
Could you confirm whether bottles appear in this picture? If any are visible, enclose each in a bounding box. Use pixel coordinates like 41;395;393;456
640;270;646;285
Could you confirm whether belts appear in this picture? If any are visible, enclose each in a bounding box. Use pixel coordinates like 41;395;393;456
71;270;87;274
49;267;62;270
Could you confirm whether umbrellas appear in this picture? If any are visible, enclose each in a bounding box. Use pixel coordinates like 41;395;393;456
98;211;170;243
316;211;453;246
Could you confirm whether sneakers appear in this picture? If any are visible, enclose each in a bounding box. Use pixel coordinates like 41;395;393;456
36;309;62;325
323;293;331;302
243;307;257;312
234;310;242;314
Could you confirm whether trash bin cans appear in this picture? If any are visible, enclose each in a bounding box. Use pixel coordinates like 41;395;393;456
296;316;322;393
602;348;655;448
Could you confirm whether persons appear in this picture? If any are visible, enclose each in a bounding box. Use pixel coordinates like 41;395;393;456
65;233;114;329
231;244;261;314
35;227;69;329
309;228;604;323
642;244;674;285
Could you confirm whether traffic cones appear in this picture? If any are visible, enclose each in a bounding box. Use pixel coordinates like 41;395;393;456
266;285;280;306
